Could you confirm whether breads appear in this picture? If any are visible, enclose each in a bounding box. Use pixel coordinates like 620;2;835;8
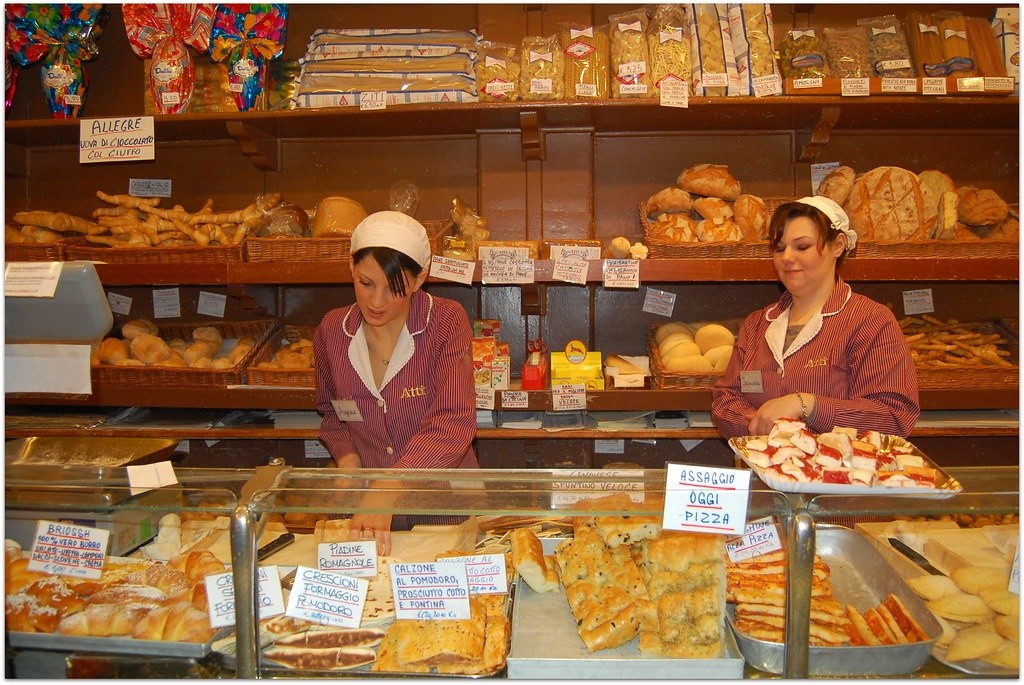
372;491;728;675
816;164;1019;242
605;353;647;374
889;512;1020;527
647;163;779;246
726;523;929;648
294;28;476;104
656;320;736;373
5;451;333;644
905;567;1020;671
90;319;317;370
747;419;939;490
442;227;605;262
882;303;1016;366
5;191;369;247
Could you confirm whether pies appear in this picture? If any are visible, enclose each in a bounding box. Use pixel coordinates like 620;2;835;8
209;554;420;670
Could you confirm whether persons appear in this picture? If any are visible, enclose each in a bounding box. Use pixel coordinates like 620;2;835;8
711;196;922;527
312;210;488;558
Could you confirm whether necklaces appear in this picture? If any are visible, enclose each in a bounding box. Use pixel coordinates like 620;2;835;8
380;357;390;365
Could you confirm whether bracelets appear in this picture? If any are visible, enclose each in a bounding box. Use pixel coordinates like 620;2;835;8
794;390;807;421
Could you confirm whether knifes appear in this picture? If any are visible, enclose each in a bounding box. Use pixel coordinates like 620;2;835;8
888;536;949;578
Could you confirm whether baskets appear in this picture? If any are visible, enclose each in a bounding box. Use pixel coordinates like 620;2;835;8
850;201;1019;258
247;324;318;386
645;317;746;389
89;318;283;388
638;194;807;258
65;234;247;263
915;316;1019;388
246;217;457;261
5;235;86;263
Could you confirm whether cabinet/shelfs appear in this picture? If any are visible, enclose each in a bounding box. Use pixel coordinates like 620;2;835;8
158;434;1020;469
5;97;1019;409
